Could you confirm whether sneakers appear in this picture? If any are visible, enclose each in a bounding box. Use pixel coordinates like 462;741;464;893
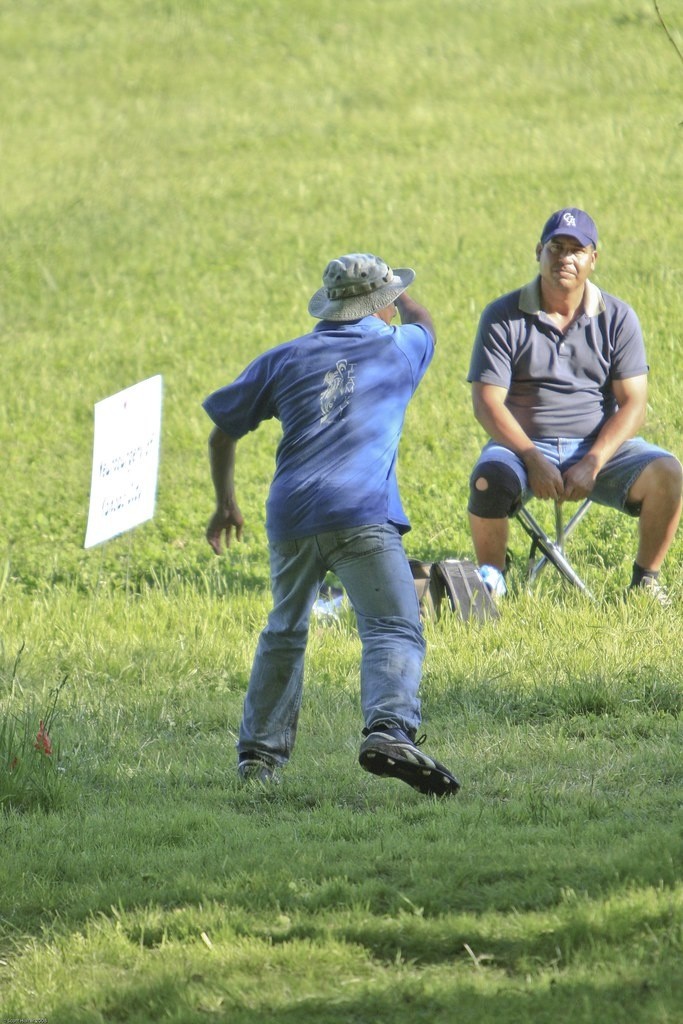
238;752;274;780
630;577;671;605
478;564;506;596
359;726;460;795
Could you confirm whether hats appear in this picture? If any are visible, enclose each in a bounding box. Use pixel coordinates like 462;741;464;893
309;254;416;320
540;208;596;247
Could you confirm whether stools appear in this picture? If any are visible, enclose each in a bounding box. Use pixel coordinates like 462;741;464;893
514;496;596;606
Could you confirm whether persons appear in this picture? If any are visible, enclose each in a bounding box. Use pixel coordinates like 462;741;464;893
465;207;683;606
200;251;463;799
